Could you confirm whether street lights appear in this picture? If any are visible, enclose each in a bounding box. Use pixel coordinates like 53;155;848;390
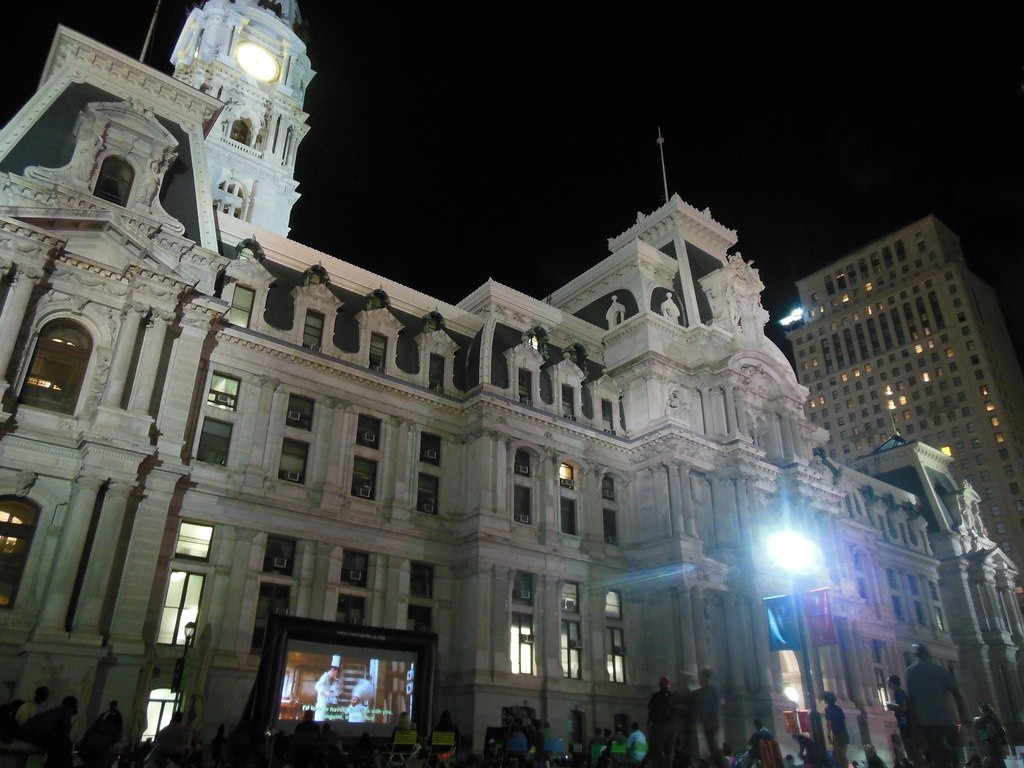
762;520;829;768
170;620;199;720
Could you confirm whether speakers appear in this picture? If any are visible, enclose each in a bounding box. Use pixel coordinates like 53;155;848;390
614;713;627;737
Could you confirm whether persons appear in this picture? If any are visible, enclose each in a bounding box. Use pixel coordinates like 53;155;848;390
2;644;1008;767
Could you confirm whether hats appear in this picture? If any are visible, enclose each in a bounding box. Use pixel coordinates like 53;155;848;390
659;678;670;689
303;710;313;719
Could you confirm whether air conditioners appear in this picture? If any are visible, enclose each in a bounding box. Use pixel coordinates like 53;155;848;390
567;415;576;421
615;646;626;654
603;490;614;497
309;345;321;352
433;386;444;393
273;607;289;616
419;627;430;632
561;479;573;486
362;433;375;442
350;618;364;625
607;429;616;436
522;635;536;643
424;450;436;458
374;367;386;374
274;559;287;568
607;535;616;544
523;400;534;406
516;465;528;473
285;472;299;481
356;487;370;498
516;514;529;523
516;590;530;599
347;570;361;580
421;504;433;513
288;411;300;421
216;394;229;403
570;640;583;648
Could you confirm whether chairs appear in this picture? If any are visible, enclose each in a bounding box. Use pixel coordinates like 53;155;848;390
591;739;649;768
381;729;417;768
424;731;455;768
544;736;565;755
288;732;349;768
502;732;528;768
218;744;249;768
158;730;185;755
